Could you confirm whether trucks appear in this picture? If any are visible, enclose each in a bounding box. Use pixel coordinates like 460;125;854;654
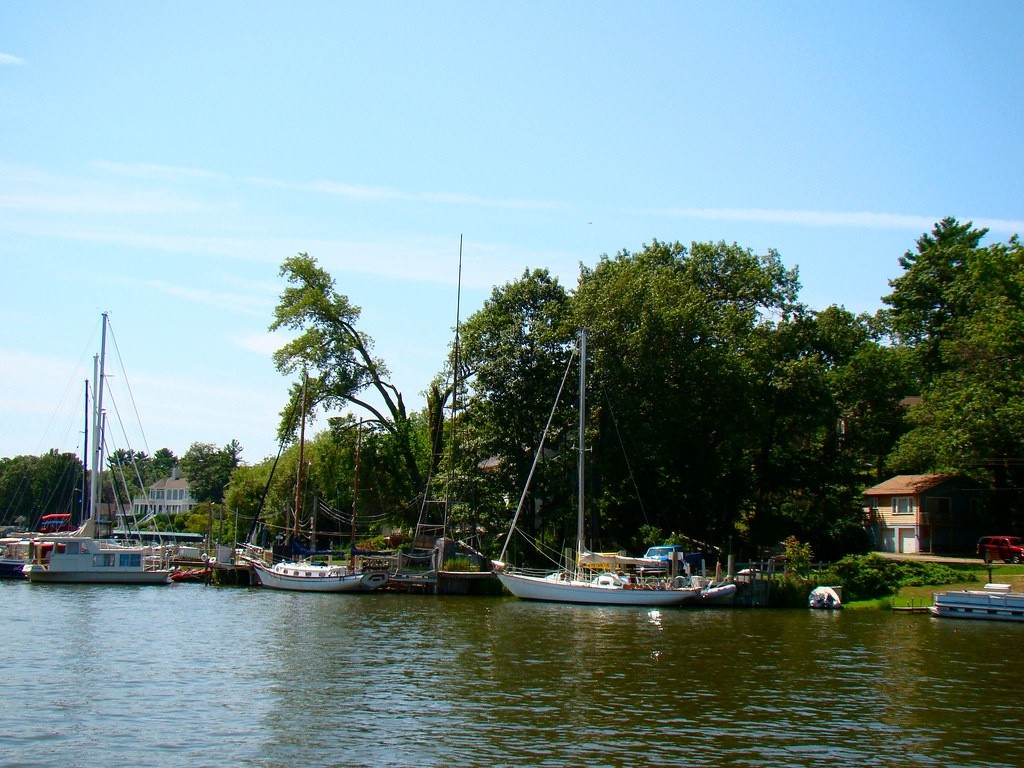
643;546;701;577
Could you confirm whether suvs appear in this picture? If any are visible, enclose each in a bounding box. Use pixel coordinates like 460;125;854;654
976;535;1024;564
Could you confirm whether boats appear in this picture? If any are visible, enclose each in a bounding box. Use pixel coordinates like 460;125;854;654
927;584;1024;622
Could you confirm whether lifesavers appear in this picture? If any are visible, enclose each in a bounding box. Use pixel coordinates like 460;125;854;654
200;553;208;561
209;556;217;564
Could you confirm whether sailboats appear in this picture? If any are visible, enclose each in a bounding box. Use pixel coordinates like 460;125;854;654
0;232;503;595
490;329;740;607
